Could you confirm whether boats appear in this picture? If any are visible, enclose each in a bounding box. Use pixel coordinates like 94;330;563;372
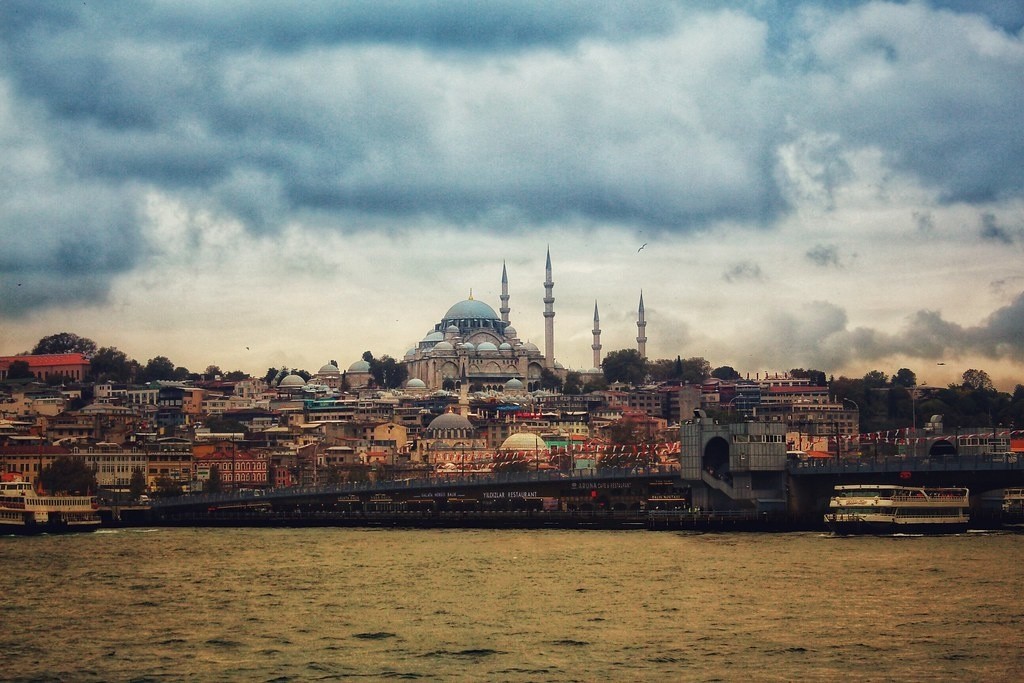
1000;489;1024;532
0;482;103;535
823;484;971;535
